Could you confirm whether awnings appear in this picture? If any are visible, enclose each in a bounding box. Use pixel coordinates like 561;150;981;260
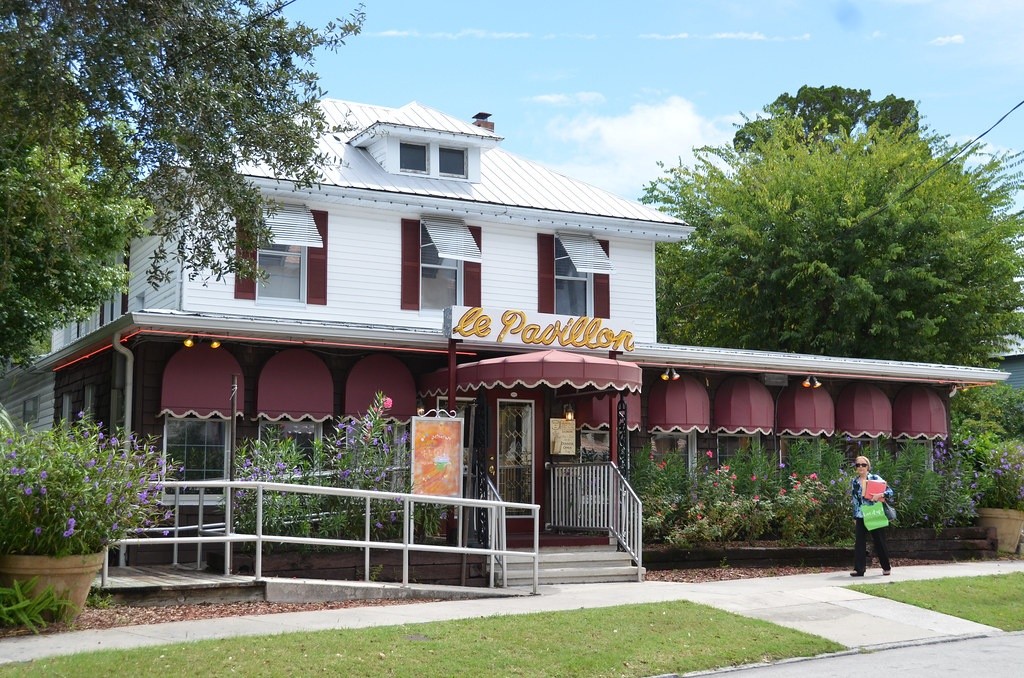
573;373;948;441
153;344;417;425
418;347;642;399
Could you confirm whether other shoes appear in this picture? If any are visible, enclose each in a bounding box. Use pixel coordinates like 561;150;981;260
850;571;863;576
883;570;890;575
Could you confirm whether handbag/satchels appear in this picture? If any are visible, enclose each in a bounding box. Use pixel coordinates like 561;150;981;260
883;501;897;520
861;500;889;531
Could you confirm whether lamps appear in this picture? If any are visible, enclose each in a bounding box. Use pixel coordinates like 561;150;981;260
564;398;576;421
184;335;195;347
210;337;221;349
416;396;425;416
672;368;680;380
661;367;671;380
803;375;810;387
813;377;822;388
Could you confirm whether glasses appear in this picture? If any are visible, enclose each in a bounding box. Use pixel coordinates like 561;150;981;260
856;463;868;467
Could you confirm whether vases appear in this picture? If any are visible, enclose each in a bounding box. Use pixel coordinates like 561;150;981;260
0;553;105;622
974;507;1023;553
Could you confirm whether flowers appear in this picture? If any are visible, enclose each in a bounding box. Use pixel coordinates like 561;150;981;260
0;406;185;558
954;432;1024;512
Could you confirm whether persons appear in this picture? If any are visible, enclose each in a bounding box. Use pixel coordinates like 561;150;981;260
851;456;893;577
